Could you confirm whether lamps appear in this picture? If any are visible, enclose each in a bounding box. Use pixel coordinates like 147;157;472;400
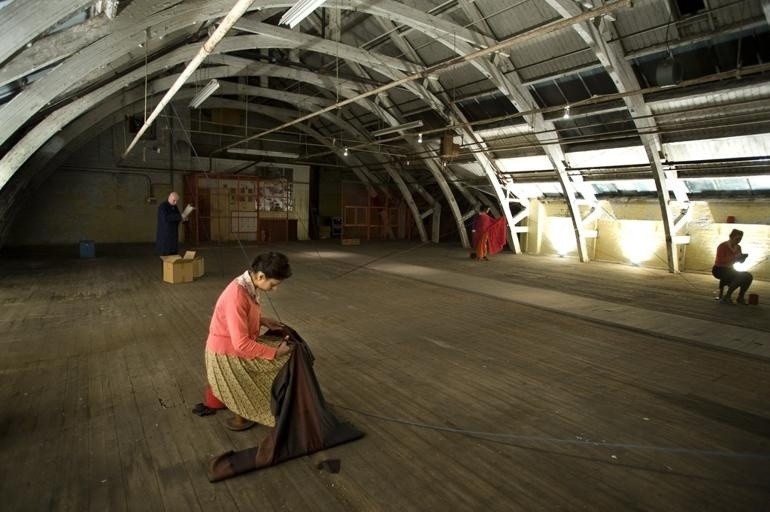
277;0;328;29
189;57;221;109
563;107;570;119
370;120;424;137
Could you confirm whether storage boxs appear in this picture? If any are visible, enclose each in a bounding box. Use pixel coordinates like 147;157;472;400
160;254;193;283
184;251;205;278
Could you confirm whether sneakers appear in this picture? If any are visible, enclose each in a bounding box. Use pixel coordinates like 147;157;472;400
736;296;749;305
722;295;735;305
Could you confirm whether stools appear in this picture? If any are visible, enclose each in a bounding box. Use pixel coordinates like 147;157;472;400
716;280;728;300
225;413;255;430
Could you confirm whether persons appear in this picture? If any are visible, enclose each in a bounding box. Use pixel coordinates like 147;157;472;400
711;230;754;305
155;191;191;262
470;204;507;258
205;250;295;427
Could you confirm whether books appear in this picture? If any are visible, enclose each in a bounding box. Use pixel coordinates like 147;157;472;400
183;202;196;218
